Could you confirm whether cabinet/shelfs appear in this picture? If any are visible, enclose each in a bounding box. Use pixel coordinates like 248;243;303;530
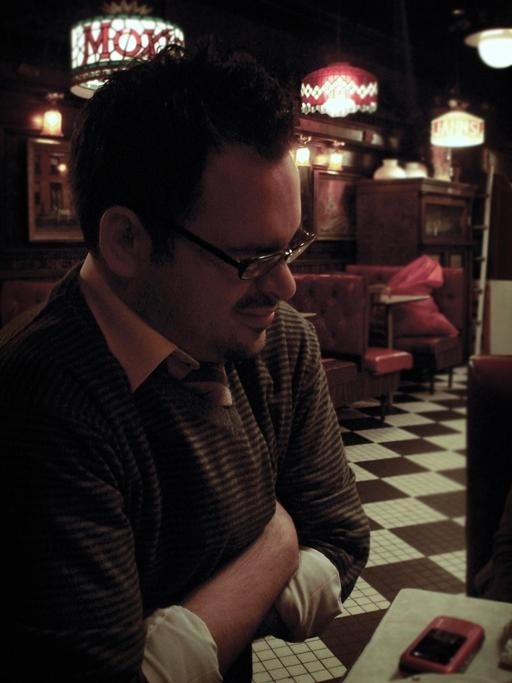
358;178;473;297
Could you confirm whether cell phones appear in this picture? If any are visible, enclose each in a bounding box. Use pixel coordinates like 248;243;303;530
401;612;485;673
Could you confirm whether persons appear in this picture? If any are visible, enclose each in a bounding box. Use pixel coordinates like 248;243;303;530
0;44;375;682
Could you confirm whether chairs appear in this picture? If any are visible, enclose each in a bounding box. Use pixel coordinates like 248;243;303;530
289;263;467;424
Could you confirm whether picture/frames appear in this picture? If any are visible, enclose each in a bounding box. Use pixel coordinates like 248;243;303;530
26;136;91;245
312;168;364;243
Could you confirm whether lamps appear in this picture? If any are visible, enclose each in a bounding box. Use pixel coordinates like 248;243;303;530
463;23;512;70
428;97;485;150
66;1;189;99
299;59;381;120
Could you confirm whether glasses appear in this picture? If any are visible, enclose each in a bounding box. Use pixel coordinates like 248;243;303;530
182;226;317;282
